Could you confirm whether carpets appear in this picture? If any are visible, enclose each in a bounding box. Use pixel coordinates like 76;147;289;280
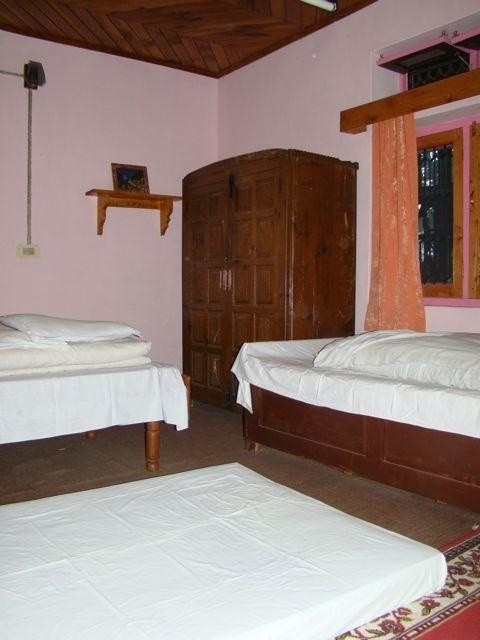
331;522;480;640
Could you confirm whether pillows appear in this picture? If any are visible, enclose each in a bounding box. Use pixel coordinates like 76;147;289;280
0;313;142;345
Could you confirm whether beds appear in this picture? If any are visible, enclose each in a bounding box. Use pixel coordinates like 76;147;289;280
0;462;448;640
241;335;480;513
0;361;181;471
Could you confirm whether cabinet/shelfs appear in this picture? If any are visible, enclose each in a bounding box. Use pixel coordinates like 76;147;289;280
181;148;359;413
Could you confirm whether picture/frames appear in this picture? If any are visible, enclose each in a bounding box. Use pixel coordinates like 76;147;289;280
110;162;150;194
